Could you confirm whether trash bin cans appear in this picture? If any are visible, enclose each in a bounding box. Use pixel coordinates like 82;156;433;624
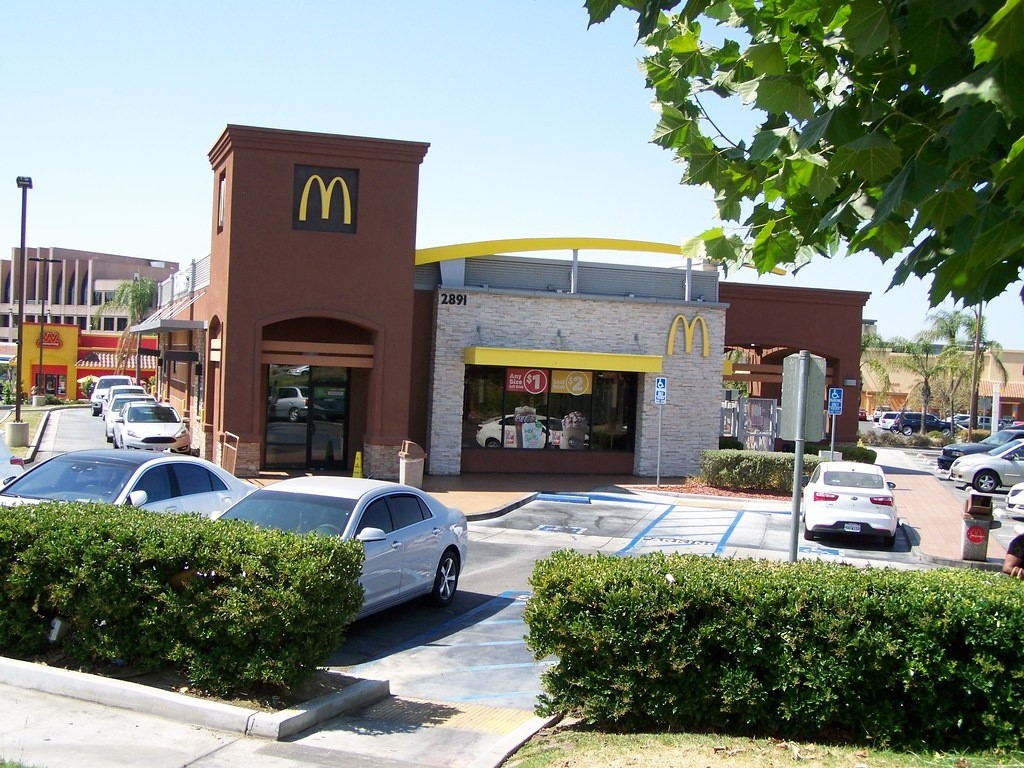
398;440;427;490
962;494;992;561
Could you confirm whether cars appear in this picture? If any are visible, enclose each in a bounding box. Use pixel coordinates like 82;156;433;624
797;459;897;546
859;408;867;420
113;402;192;457
267;384;327;421
104;393;158;443
1002;417;1024;430
287;364;310;377
104;385;146;420
190;473;470;632
0;450;258;529
473;412;593;450
949;437;1024;492
874;406;892;422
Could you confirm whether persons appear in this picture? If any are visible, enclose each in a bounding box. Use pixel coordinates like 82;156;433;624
1002;532;1024;579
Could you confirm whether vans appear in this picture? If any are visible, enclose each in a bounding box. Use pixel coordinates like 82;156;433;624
943;414;972;427
936;429;1024;471
975;417;1007;430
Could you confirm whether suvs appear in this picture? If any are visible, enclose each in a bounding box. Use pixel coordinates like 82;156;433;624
89;375;133;416
894;412;958;436
881;412;900;434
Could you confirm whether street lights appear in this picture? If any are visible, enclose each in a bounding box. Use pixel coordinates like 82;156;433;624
28;256;62;406
6;175;35;448
